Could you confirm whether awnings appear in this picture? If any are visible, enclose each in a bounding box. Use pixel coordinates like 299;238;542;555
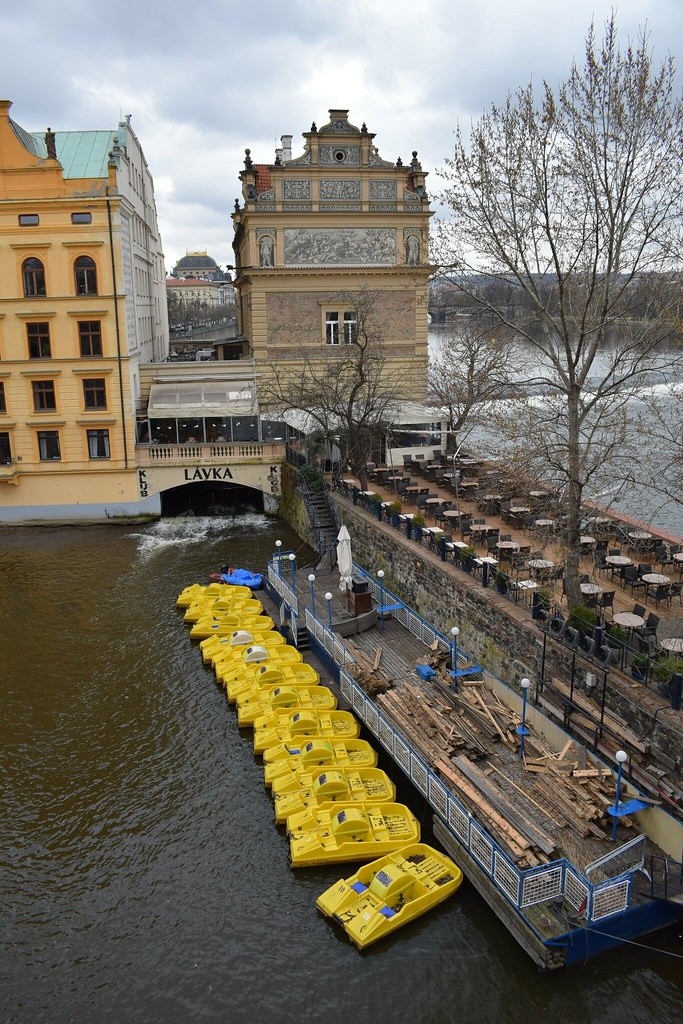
145;379;261;418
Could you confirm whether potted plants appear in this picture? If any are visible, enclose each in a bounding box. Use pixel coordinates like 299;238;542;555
629;652;650;680
653;655;683;701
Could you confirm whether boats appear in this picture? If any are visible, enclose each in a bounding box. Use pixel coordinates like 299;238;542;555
262;740;378;790
268;767;397;826
221;662;321;705
211;644;304;684
198;630;287;665
184;599;264;624
313;844;464;953
253;710;363;755
234;685;338;728
209;564;264;590
175;582;253;609
188;614;276;640
286;801;422;868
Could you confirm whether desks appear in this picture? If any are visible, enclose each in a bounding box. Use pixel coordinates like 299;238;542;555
358;491;375;497
426;465;443;469
348;589;372;617
445;541;469;558
426;498;445;516
529;491;549;498
514;579;540;603
613;612;644;642
372;468;392;472
341;479;355;484
443;510;465;527
381;502;395;508
508;506;531;513
482;494;502;500
496;541;520;560
641;573;670;596
398;513;416;522
672;553;683;565
443;473;460;478
459;482;479;488
412;459;427;469
628;531;652;544
473;556;499;579
568;583;604;602
498;478;516;484
405;486;424;497
604;556;631;577
340;448;683;659
528;559;556;578
421;527;444;536
534;519;555;526
587;517;610;523
469;524;492;542
660;637;683;658
579;536;596;545
387;476;405;482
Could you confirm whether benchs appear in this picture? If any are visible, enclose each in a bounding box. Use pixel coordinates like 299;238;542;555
328;608;378;638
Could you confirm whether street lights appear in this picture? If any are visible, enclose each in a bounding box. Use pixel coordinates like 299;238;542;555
608;750;628;844
288;553;296;593
516;678;531;760
324;592;332;633
448;627;460;693
308;573;315;616
377;569;385;632
275;540;282;576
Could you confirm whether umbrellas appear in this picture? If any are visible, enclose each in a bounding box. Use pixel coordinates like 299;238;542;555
336;524;353;614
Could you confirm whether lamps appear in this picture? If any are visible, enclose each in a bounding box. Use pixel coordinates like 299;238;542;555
226;265;249;270
536;609;551;628
548;617;568;637
593;644;619;668
561;626;580;648
575;635;597;658
